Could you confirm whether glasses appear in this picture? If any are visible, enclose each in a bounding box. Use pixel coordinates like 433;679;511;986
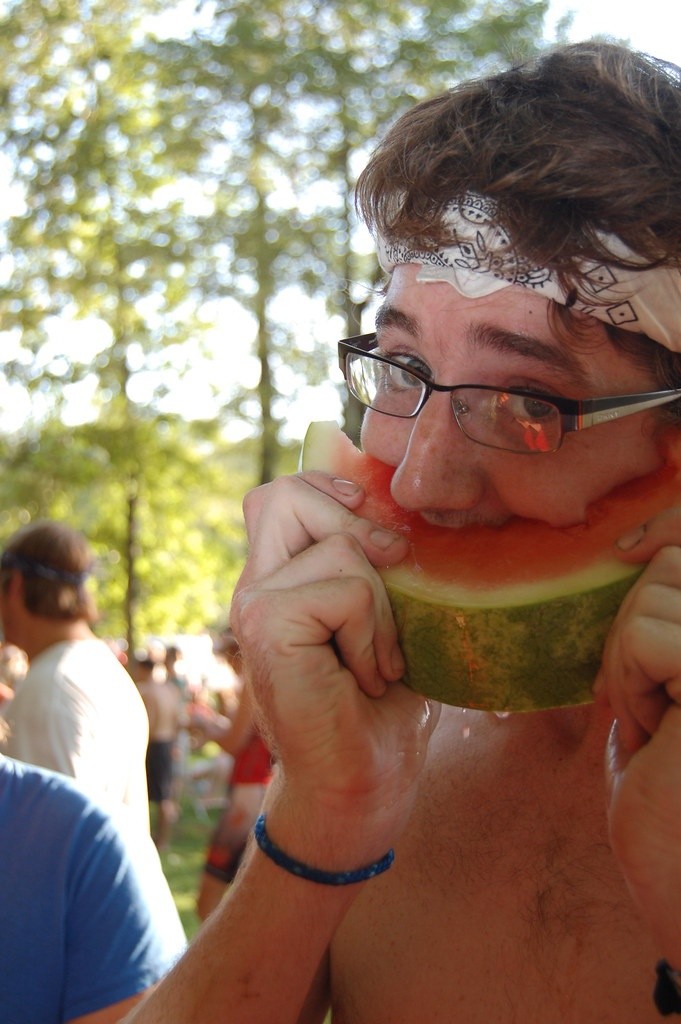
337;332;681;455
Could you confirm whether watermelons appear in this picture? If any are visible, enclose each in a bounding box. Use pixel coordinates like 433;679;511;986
299;415;681;713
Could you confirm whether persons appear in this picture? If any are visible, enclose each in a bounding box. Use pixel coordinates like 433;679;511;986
108;40;681;1024
0;524;275;1021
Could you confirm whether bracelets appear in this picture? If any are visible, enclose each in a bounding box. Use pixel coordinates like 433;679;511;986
254;807;400;887
651;958;681;1015
